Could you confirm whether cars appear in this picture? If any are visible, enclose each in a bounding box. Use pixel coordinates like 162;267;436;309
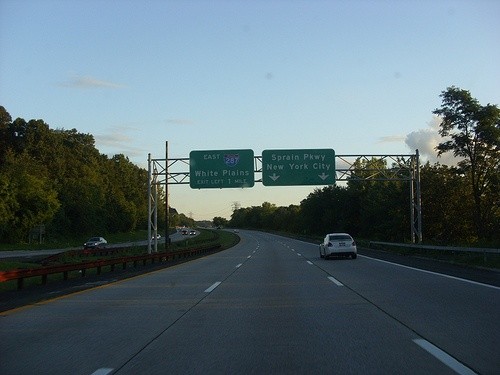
152;234;162;240
319;232;358;261
83;237;108;250
176;226;240;236
